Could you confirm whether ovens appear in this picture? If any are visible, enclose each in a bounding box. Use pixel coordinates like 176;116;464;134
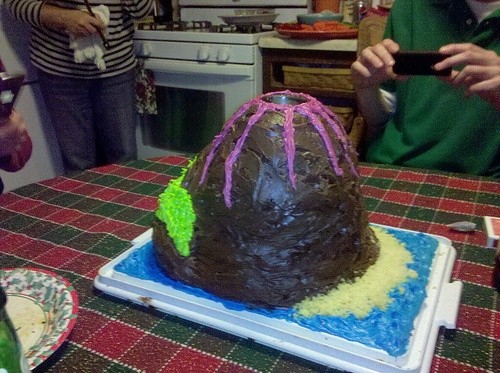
131;59;266;160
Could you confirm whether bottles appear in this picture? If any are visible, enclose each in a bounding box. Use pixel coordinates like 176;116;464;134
0;286;21;372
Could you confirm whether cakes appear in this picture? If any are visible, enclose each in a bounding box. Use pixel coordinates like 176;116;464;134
114;90;439;357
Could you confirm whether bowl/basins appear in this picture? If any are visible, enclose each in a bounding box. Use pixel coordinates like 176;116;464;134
235;9;274;16
297;13;344;24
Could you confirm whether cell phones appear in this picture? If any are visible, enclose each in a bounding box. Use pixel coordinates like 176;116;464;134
0;69;26;125
389;49;453;80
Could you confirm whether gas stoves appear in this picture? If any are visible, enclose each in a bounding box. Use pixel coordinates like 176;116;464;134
132;20;278;44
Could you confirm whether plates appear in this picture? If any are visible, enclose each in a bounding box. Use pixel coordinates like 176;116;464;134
220;14;279;24
0;266;79;372
275;26;359;38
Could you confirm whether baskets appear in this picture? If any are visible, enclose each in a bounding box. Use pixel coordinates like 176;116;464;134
325;103;355;134
282;66;355;91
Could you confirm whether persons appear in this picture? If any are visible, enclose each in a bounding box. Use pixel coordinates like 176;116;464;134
0;59;32;195
0;0;154;175
350;0;500;179
